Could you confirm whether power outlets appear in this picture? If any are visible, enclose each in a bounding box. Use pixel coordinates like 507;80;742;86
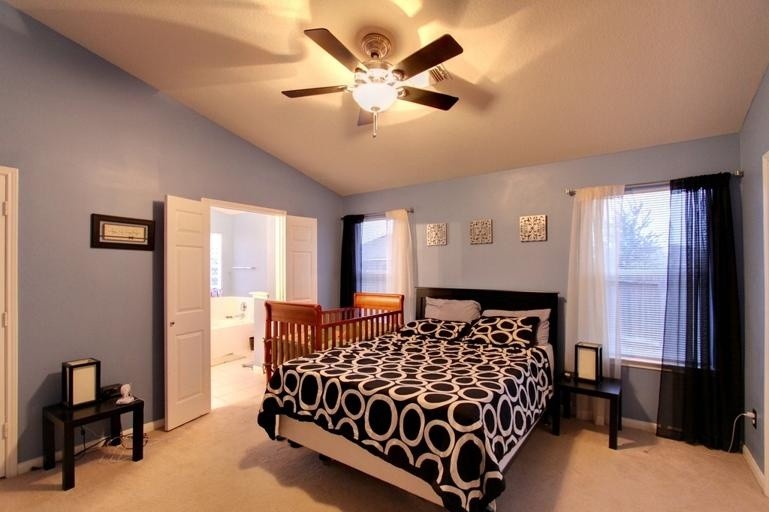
751;408;758;430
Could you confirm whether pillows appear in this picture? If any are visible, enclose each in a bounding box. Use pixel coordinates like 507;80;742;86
485;306;551;350
423;294;484;330
400;316;467;346
468;314;537;350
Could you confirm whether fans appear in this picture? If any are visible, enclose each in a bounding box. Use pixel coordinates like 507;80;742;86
280;24;465;128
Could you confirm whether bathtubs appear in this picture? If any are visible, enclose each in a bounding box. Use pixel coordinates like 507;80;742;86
210;291;253;367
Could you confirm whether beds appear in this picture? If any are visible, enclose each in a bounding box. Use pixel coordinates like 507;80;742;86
260;291;405;396
256;284;564;512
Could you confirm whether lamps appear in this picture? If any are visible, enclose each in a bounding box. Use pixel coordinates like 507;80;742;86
573;342;603;385
60;357;102;410
344;63;408;139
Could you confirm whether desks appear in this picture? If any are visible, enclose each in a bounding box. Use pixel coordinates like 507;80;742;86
41;391;144;491
552;370;625;453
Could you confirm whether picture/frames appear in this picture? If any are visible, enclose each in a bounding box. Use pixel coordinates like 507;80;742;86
88;211;156;254
